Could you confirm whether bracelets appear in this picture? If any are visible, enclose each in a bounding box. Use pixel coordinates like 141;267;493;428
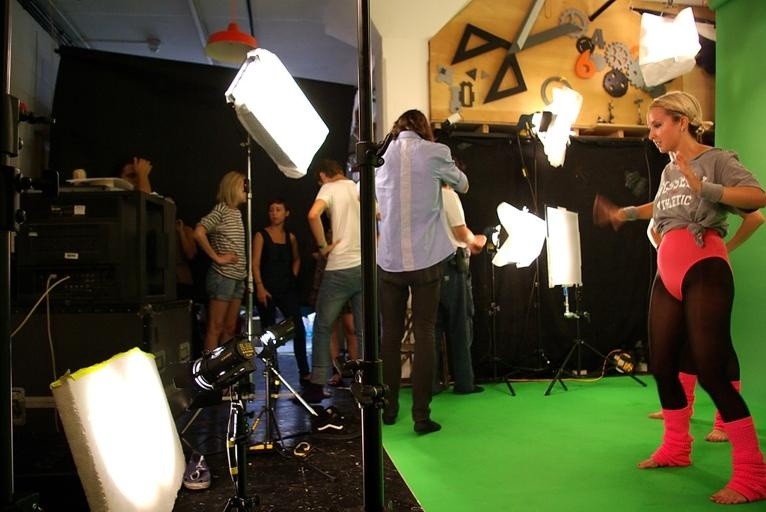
254;278;262;285
464;231;476;245
699;180;725;203
317;240;328;250
620;204;641;223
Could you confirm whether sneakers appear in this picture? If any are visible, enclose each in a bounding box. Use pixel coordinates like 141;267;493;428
316;418;361;440
182;455;212;491
310;406;353;434
222;387;239;402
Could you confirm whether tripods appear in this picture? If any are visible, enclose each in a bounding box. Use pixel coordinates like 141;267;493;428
508;255;569;392
543;285;648;397
473;252;524;397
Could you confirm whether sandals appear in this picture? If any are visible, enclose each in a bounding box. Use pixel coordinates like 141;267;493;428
328;372;343;386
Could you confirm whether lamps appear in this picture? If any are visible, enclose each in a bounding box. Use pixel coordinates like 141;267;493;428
203;1;258;65
191;319;321;512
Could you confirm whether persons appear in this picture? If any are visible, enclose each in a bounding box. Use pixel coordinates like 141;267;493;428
646;138;766;443
194;170;249;353
591;89;766;505
310;209;360;386
113;156;154;195
374;110;470;432
439;155;487;395
251;196;324;399
291;157;381;405
160;192;196;300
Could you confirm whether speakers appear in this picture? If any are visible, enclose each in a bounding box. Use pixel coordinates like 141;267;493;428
0;189;179;312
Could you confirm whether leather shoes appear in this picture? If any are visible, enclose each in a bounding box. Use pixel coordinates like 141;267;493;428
300;372;312;387
293;390;325;404
270;380;281;394
